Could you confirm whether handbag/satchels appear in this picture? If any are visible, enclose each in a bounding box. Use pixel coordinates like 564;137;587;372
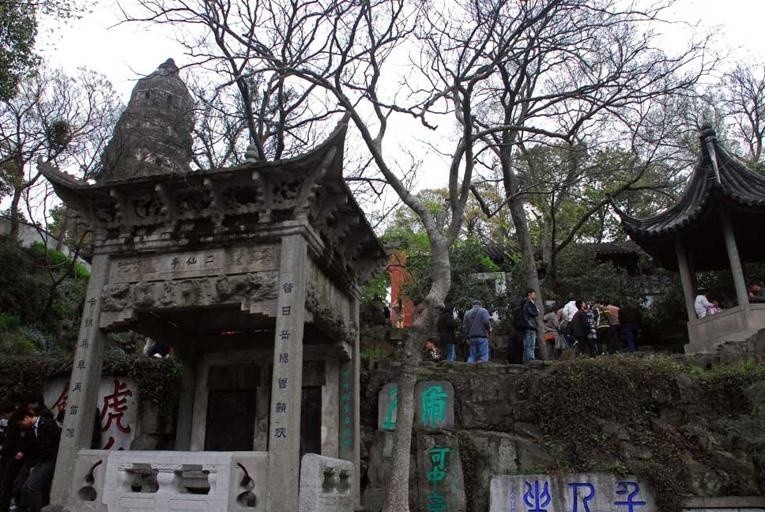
554;335;567;350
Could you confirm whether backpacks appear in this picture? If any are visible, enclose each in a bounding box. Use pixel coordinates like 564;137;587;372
510;299;537;332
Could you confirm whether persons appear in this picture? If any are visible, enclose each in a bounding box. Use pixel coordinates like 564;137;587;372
543;294;640;359
694;288;721;320
747;281;764;304
506;288;541;363
391;292;403;329
0;394;102;512
426;300;490;364
